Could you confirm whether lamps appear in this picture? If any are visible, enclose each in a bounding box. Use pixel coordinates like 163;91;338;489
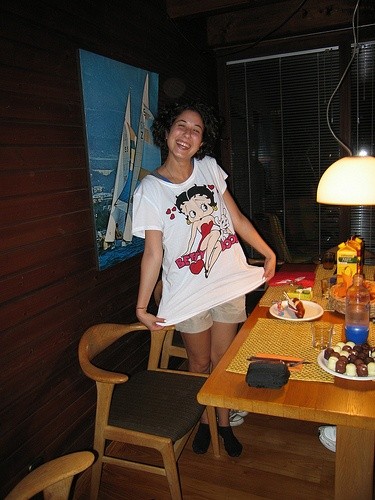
316;0;375;206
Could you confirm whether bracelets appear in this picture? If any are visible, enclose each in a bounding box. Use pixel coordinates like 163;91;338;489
136;307;148;310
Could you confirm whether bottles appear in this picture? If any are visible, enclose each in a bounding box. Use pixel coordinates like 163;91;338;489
337;237;362;282
345;273;370;345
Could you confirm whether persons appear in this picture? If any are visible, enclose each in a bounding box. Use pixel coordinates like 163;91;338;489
131;98;277;458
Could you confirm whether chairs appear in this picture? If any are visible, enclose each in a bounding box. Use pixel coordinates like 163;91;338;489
4;451;95;500
153;281;188;370
270;215;295;263
246;258;285;316
78;322;220;500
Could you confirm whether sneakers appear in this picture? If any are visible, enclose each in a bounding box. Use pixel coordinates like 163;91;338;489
216;411;244;426
229;409;248;416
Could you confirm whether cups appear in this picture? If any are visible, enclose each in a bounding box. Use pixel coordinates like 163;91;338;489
311;320;333;349
323;251;335;270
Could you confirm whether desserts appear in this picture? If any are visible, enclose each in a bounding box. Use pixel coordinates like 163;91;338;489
323;342;375;377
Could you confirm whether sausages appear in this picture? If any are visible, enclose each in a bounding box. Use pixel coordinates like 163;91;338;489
292;298;305;318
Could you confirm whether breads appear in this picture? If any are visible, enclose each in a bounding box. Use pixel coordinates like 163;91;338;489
333;274;375;303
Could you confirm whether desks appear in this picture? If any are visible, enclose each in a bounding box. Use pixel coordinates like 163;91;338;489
197;263;375;500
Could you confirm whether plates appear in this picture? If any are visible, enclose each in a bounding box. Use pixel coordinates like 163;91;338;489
318;349;375;381
269;300;324;322
